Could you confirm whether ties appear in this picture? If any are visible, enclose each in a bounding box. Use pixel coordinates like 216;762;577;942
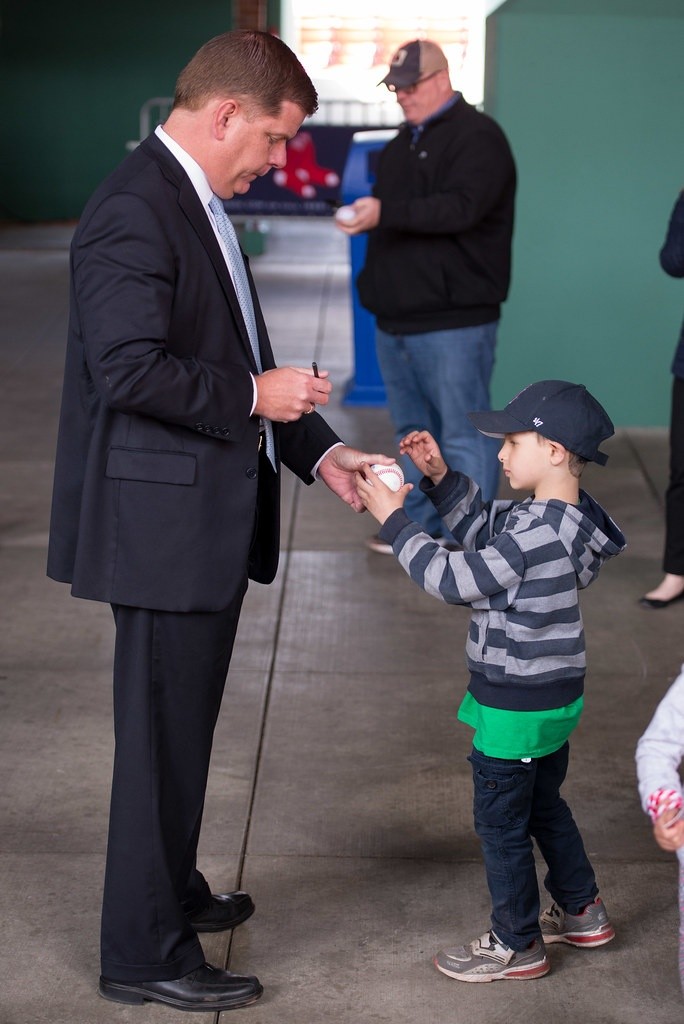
209;193;276;473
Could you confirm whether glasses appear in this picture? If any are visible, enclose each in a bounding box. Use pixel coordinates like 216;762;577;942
385;72;436;94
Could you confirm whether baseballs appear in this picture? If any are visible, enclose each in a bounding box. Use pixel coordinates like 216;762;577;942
366;462;404;491
335;206;355;222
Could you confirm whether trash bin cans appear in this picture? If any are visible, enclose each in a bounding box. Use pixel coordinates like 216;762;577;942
342;128;400;406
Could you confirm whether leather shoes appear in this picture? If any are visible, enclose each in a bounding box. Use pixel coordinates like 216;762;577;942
186;889;255;933
97;962;264;1011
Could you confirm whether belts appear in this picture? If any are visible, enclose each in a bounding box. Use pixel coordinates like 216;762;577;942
258;433;266;454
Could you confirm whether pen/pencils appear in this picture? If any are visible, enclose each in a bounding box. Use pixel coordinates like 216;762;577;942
312;361;320;380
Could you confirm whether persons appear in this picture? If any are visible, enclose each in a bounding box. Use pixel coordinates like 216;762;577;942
633;660;684;894
351;380;628;984
45;30;395;1014
335;40;517;559
639;190;683;612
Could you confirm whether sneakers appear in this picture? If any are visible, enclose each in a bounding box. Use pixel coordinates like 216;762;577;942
539;897;615;947
434;928;550;982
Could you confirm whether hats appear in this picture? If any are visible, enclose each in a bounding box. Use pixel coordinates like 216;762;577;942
375;39;448;88
466;380;615;466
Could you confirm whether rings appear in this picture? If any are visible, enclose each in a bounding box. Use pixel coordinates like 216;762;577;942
304;402;318;416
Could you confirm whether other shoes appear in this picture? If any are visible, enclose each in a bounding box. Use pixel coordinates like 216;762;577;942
368;533;447;555
637;588;684;611
